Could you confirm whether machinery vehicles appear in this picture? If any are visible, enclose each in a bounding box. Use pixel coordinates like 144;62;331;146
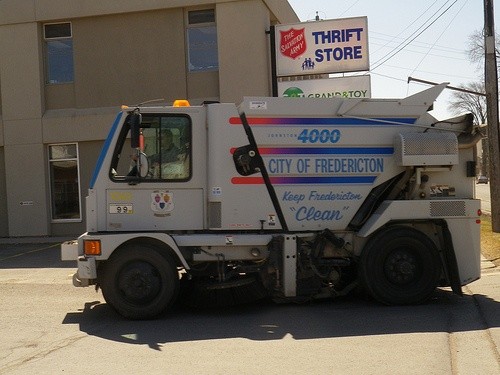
59;81;483;322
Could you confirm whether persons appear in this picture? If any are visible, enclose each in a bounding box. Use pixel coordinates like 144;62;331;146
142;128;180;179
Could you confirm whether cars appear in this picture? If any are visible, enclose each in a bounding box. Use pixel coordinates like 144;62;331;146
478;175;488;184
